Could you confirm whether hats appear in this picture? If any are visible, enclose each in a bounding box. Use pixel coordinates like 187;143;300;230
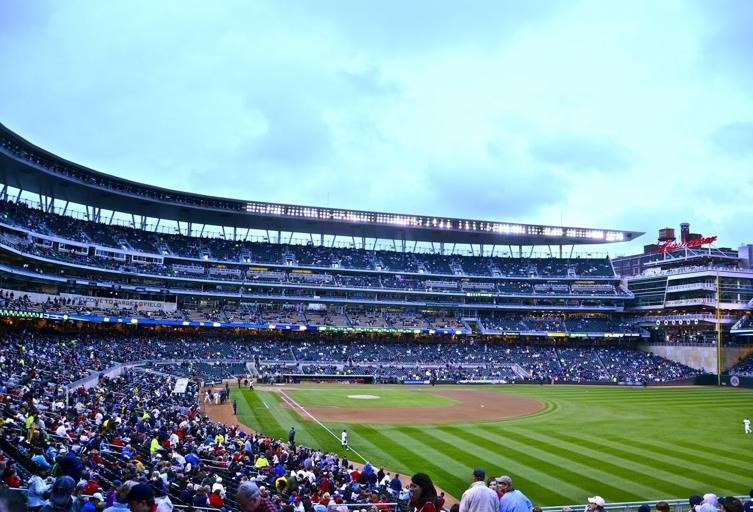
473;469;484;476
694;503;717;512
50;475;75;505
494;476;512;485
588;496;605;506
93;492;104;502
120;483;154;504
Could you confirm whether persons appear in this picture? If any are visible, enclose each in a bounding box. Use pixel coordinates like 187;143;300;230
0;389;298;511
340;428;349;452
740;416;752;435
298;444;752;512
0;194;752;391
